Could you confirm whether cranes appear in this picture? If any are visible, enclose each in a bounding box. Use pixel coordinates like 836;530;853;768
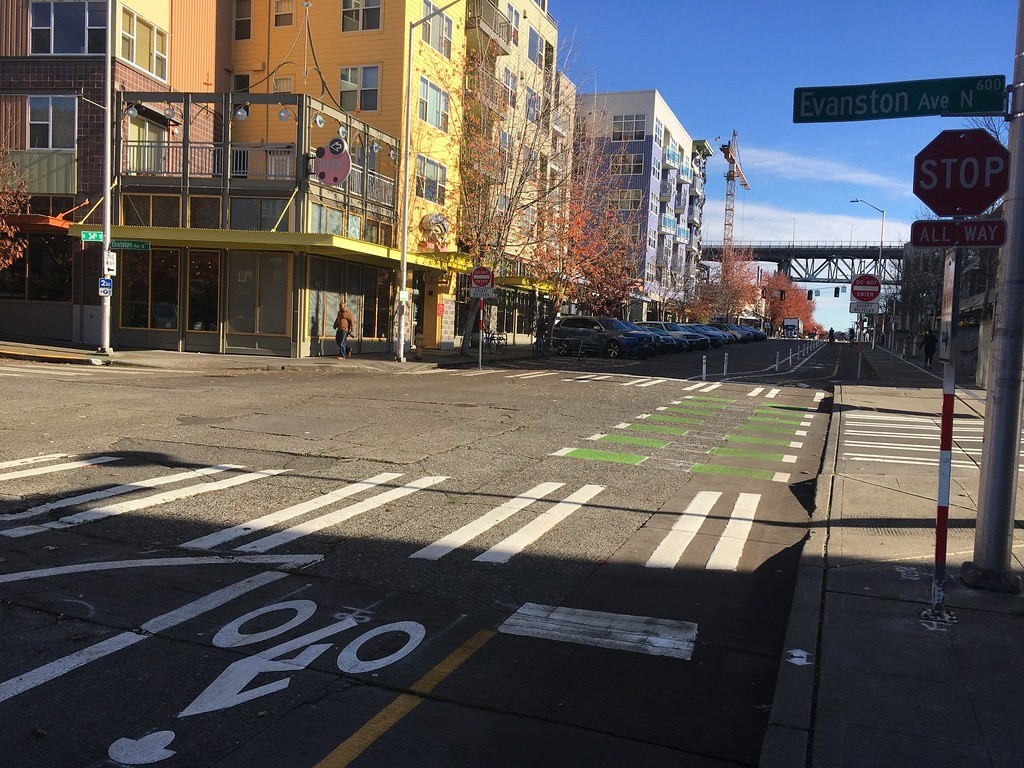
718;129;752;282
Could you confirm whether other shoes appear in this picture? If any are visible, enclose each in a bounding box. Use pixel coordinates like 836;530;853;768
337;356;345;360
347;347;352;358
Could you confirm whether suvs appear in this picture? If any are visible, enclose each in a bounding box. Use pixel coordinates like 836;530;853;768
717;324;753;344
633;322;699;350
552;316;655;358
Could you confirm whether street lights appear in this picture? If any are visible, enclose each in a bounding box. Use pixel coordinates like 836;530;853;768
850;200;884;351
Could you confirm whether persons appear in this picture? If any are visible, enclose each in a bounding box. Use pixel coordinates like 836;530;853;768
849;329;855;344
333;303;354;359
828;328;835;343
918;330;939;369
535;311;547;349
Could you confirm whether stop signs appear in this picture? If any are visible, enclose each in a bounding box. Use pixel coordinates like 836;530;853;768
471;267;493;288
909;129;1012;220
850;275;882;302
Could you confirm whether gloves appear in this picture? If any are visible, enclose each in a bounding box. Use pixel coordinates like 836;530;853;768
334;323;337;329
350;331;353;337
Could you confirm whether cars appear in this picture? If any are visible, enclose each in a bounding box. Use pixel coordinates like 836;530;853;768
706;326;736;345
677;324;724;347
746;325;767;341
646;327;689;350
621;321;665;349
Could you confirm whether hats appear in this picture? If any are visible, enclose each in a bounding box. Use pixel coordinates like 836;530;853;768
339;303;345;308
